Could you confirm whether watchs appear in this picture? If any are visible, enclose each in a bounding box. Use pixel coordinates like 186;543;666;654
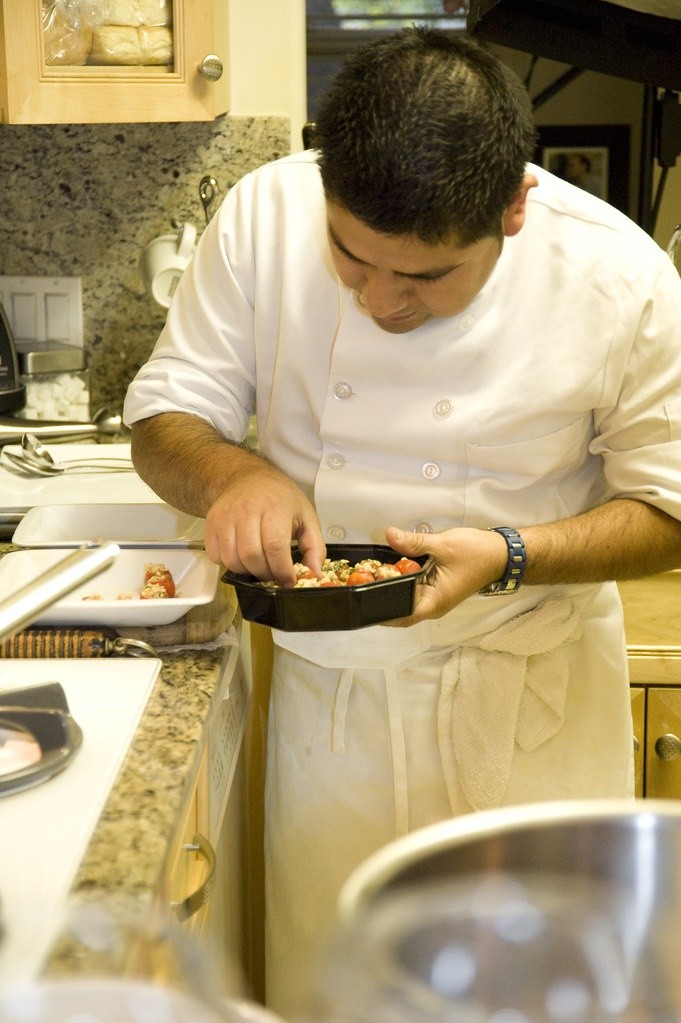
477;527;526;596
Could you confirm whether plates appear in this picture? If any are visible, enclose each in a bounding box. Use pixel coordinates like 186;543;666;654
13;504;206;548
0;547;221;627
0;444;168;521
221;545;432;633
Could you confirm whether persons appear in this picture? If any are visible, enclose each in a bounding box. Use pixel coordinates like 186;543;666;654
122;25;681;1023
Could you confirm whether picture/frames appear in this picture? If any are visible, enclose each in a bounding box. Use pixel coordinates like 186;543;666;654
530;123;631;213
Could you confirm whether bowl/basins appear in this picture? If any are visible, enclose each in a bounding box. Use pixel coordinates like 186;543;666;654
339;799;680;1023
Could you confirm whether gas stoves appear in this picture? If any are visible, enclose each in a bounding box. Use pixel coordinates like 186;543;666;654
0;657;159;997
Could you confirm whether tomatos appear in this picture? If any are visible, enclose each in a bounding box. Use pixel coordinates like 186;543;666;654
140;566;175;599
296;560;421;588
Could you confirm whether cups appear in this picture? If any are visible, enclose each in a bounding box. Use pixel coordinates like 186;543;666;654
139;222;196;307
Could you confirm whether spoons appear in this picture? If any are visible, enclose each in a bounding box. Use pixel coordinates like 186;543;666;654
4;452;135;477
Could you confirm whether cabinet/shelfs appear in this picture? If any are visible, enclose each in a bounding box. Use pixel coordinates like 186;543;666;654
633;685;681;799
1;1;231;125
